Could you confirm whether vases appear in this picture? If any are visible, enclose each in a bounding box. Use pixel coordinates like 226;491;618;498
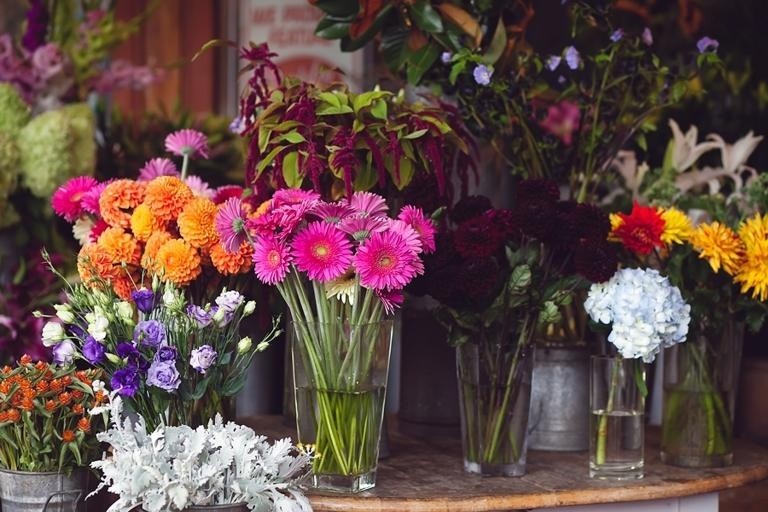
1;310;768;512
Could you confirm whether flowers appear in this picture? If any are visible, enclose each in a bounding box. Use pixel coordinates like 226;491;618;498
0;0;767;470
